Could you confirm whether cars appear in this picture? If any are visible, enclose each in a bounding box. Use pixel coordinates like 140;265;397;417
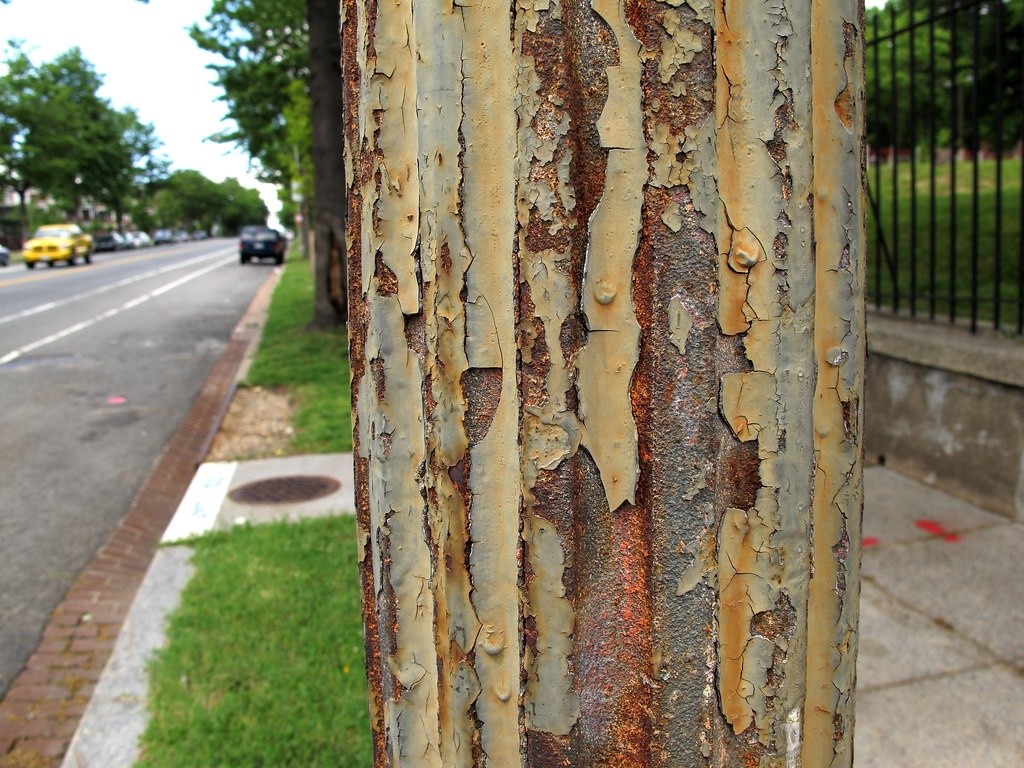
23;223;94;268
0;244;9;267
238;224;286;265
97;228;208;250
